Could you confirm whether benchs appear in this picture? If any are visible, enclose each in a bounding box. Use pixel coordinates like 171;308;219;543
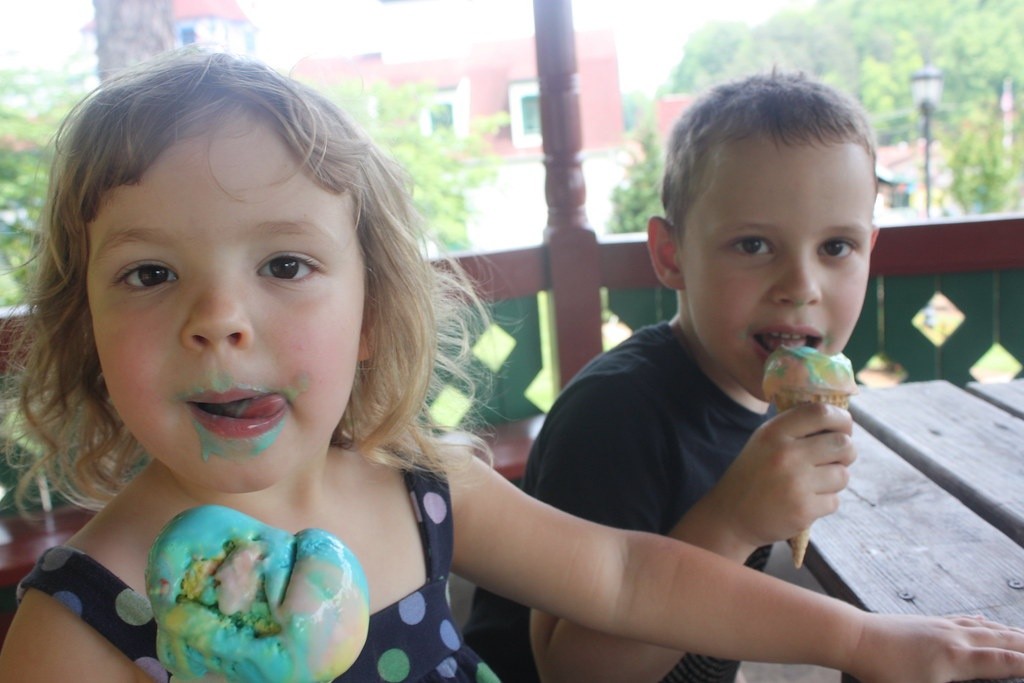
0;415;547;589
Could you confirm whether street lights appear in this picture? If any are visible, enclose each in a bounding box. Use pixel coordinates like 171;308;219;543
910;62;942;216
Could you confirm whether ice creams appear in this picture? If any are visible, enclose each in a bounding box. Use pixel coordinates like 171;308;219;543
761;345;859;568
144;503;369;683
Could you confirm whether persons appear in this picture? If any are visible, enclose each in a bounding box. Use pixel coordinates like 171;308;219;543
0;42;1024;683
463;63;876;683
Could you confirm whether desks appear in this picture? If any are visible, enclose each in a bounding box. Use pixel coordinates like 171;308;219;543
796;378;1024;683
